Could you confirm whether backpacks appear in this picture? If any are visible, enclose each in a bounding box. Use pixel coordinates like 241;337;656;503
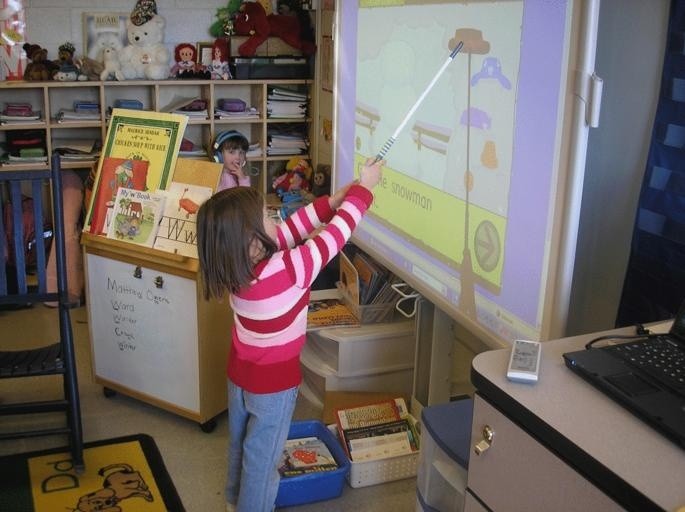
5;195;53;276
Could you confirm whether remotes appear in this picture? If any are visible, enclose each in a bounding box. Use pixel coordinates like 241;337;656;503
507;340;542;384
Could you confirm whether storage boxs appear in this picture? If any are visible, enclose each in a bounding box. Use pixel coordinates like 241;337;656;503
228;36;304;58
416;398;474;512
231;58;307;79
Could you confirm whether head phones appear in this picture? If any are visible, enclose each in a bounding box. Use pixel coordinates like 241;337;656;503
208;129;241;163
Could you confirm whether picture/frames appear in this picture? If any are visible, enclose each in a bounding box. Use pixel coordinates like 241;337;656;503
198;42;215;67
82;12;130;62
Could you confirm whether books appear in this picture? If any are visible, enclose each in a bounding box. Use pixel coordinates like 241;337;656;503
161;87;308;158
0;109;112;167
305;249;409;333
276;397;421;480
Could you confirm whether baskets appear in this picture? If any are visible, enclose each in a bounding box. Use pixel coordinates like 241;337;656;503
326;423;420;489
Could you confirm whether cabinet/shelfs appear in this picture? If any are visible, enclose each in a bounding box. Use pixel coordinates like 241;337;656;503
80;231;235;433
299;308;415;409
465;318;684;512
0;78;316;208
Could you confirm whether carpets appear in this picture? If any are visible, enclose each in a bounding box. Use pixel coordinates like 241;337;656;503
1;434;189;512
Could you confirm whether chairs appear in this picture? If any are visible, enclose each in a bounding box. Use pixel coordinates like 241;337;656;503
0;150;86;474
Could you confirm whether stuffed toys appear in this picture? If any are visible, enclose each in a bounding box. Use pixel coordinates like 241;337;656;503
23;0;241;82
234;0;318;58
271;159;331;205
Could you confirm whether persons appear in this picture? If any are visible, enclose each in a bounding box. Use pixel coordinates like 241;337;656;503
212;129;251;195
197;158;385;512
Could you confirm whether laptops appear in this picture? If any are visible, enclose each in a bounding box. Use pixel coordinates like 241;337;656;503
563;296;685;451
